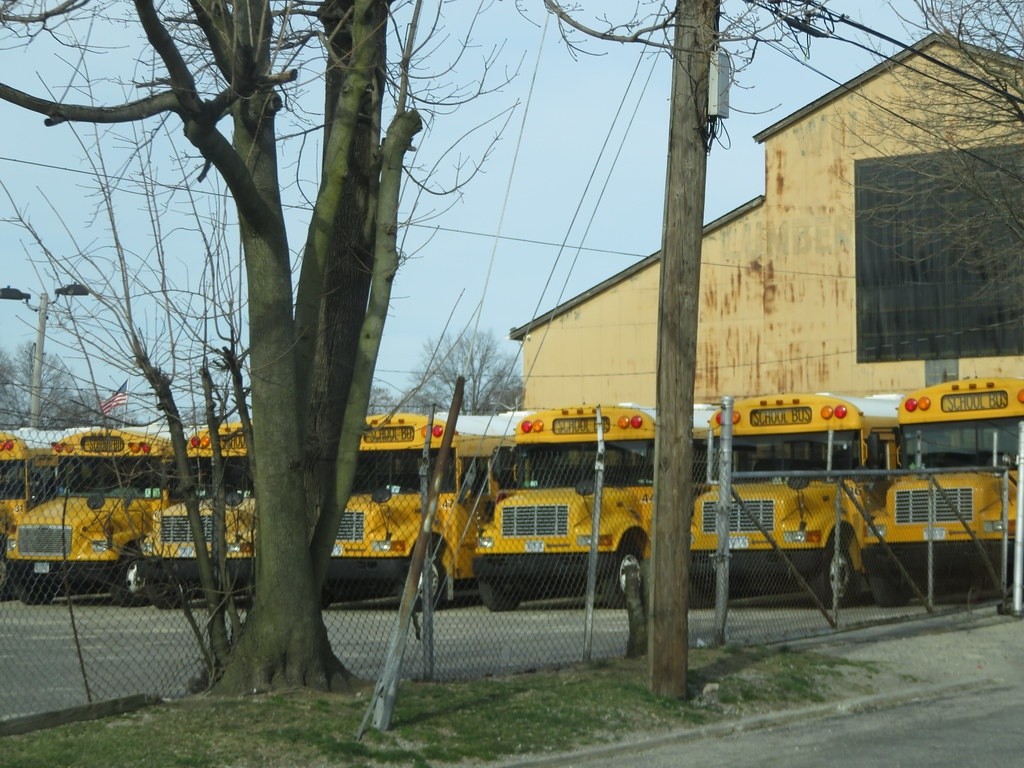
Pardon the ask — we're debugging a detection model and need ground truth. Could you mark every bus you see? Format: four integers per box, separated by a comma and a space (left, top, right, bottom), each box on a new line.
861, 376, 1023, 608
322, 411, 546, 611
6, 425, 199, 605
688, 392, 908, 608
145, 418, 255, 613
0, 427, 60, 602
472, 399, 723, 611
